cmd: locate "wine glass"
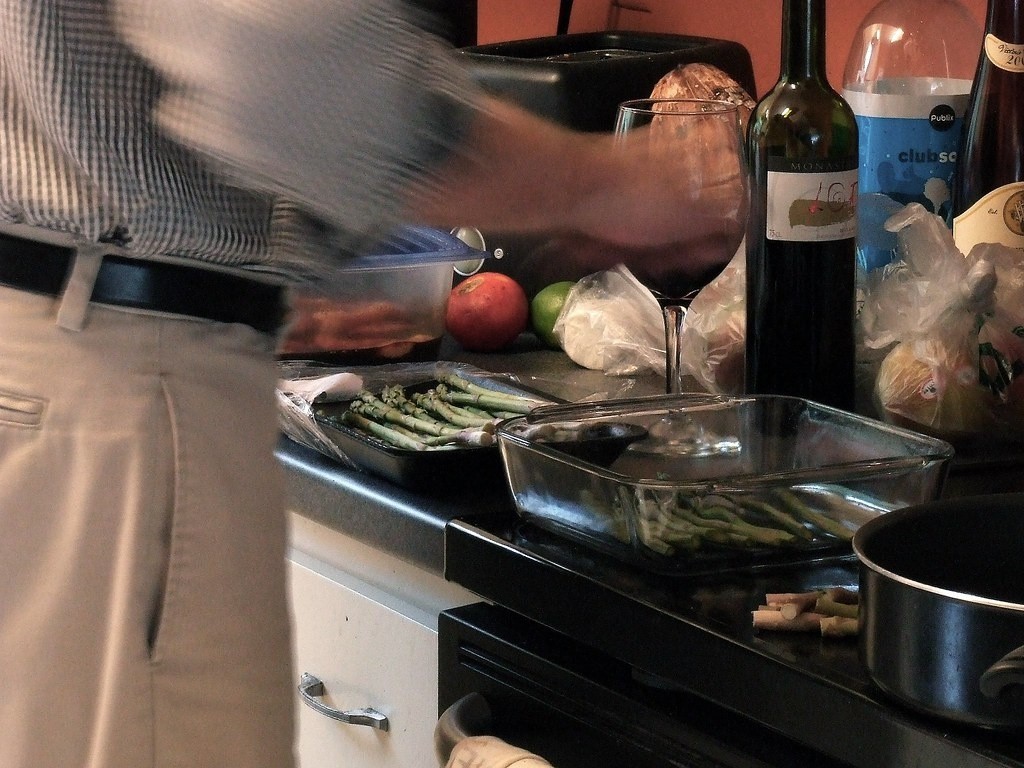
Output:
[608,98,749,460]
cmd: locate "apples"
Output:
[441,272,528,351]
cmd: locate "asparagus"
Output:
[340,373,610,453]
[752,587,861,637]
[575,485,855,555]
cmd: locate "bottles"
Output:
[837,0,994,368]
[745,0,860,440]
[951,0,1024,236]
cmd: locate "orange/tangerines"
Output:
[530,281,580,348]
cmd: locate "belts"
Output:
[0,231,287,334]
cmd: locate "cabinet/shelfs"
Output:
[285,549,437,766]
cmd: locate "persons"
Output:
[0,0,748,768]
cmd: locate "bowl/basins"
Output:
[851,492,1024,734]
